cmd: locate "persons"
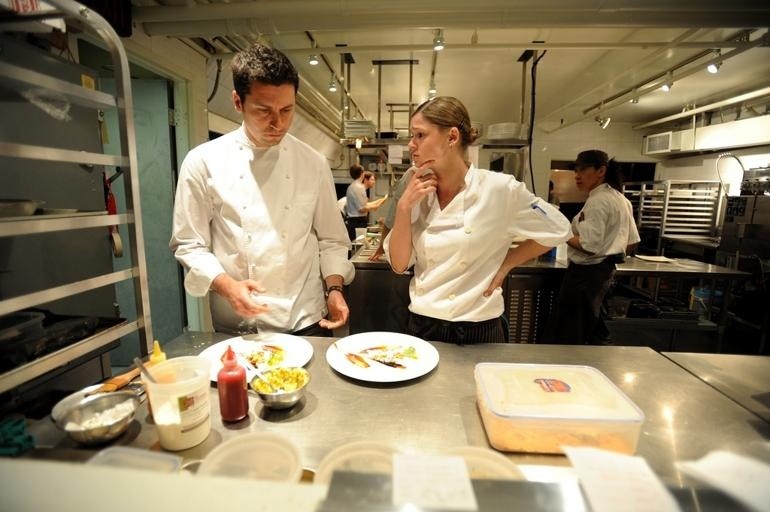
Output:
[166,43,356,341]
[336,163,390,241]
[549,150,641,345]
[382,95,575,345]
[548,179,561,211]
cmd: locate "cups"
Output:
[315,441,412,484]
[196,431,304,484]
[443,445,527,480]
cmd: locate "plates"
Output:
[42,208,79,215]
[51,384,148,422]
[635,254,675,262]
[196,333,314,384]
[325,331,441,382]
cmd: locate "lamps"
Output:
[309,41,338,94]
[592,115,612,131]
[434,27,447,51]
[662,50,728,91]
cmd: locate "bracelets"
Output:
[325,286,343,297]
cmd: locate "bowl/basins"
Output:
[249,366,311,410]
[1,198,46,217]
[57,391,141,446]
[364,226,384,241]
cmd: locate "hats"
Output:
[563,150,608,170]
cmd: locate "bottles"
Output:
[216,344,248,422]
[145,340,168,419]
